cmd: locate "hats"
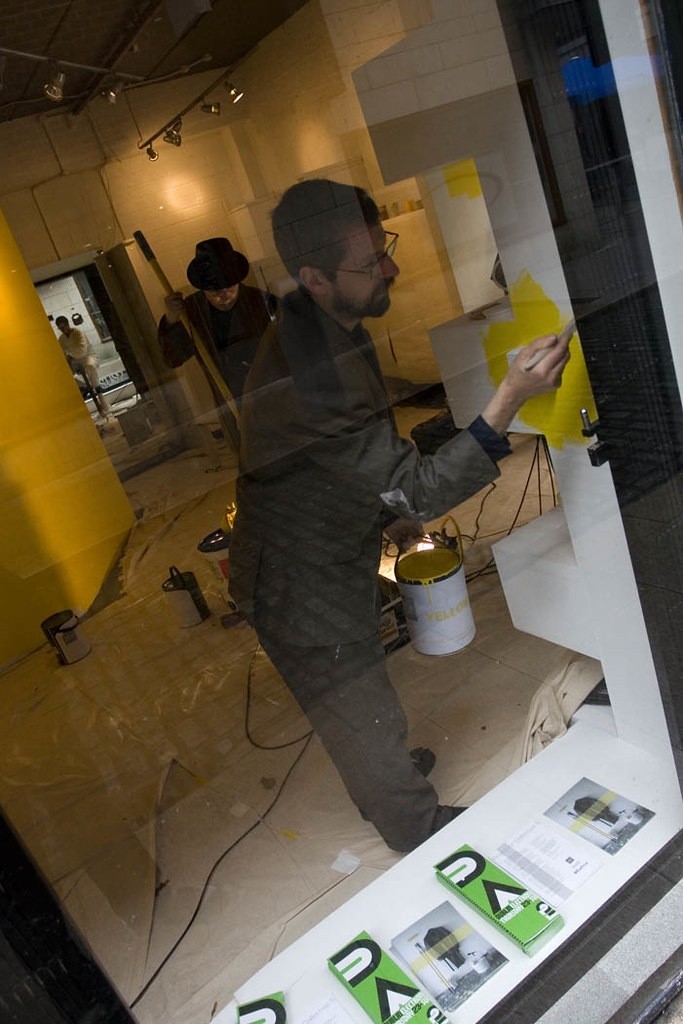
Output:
[186,237,250,292]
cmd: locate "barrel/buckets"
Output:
[197,528,238,614]
[162,565,210,628]
[40,609,94,665]
[395,534,475,657]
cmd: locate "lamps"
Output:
[41,59,66,103]
[102,77,123,104]
[122,45,259,161]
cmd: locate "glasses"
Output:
[321,230,399,280]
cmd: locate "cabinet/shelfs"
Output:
[354,0,683,795]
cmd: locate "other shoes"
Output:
[358,746,436,821]
[98,394,110,412]
[75,379,86,388]
[449,806,469,821]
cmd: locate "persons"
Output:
[54,316,104,395]
[157,238,281,451]
[227,177,573,852]
